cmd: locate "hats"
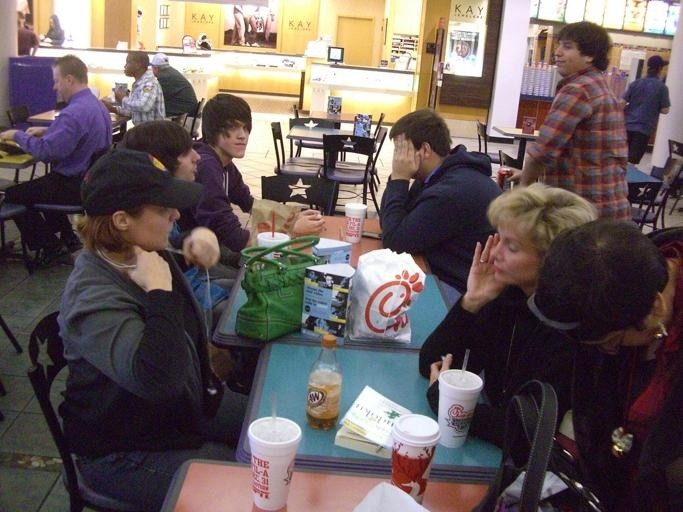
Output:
[146,53,169,66]
[80,148,206,216]
[648,55,669,66]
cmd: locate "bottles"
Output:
[306,334,344,430]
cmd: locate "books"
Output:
[334,383,421,464]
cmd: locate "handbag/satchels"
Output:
[236,236,324,343]
[470,379,604,511]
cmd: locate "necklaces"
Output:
[94,245,215,378]
[610,344,657,463]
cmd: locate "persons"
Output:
[38,14,65,46]
[230,4,248,47]
[376,107,505,293]
[137,10,143,33]
[148,53,201,136]
[17,10,39,57]
[99,50,167,126]
[415,180,600,452]
[523,215,683,511]
[245,12,276,48]
[121,118,259,395]
[166,93,326,387]
[506,20,632,223]
[57,145,236,512]
[620,55,671,167]
[0,52,115,272]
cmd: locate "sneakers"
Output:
[59,233,83,252]
[26,241,70,270]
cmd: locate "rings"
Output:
[480,260,487,263]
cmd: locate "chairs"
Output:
[271,104,388,218]
[627,179,664,230]
[635,165,683,228]
[666,139,683,167]
[26,312,149,511]
[171,97,206,140]
[5,105,33,129]
[262,175,339,215]
[32,143,118,262]
[499,150,522,172]
[0,197,30,352]
[475,119,499,164]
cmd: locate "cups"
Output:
[438,368,485,449]
[246,416,302,511]
[344,202,367,244]
[391,414,442,505]
[257,231,291,262]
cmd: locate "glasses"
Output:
[595,333,626,355]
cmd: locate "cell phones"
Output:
[362,230,383,240]
